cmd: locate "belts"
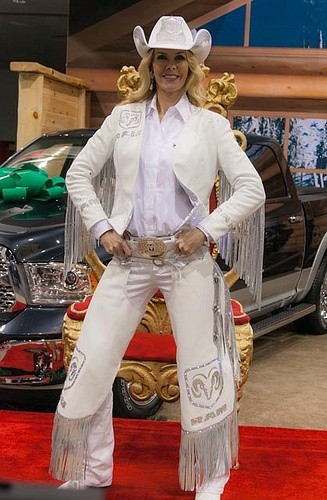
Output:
[125,239,177,258]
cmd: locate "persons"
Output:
[52,13,267,500]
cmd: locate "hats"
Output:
[133,16,212,60]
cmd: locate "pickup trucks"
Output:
[1,127,327,419]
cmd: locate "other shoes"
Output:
[195,492,220,500]
[57,478,91,492]
